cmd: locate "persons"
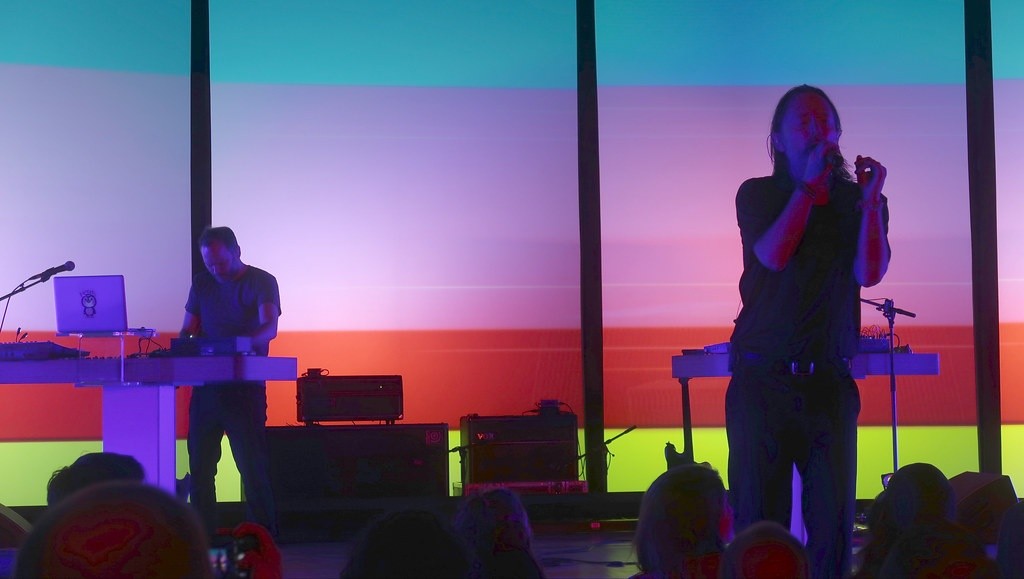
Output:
[629,464,811,579]
[854,463,1024,579]
[727,86,891,579]
[343,489,546,579]
[16,452,282,579]
[184,227,280,536]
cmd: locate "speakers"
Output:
[947,472,1020,544]
[266,422,449,546]
[460,415,579,488]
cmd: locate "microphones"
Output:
[826,151,844,167]
[30,261,75,280]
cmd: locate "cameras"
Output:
[208,534,258,579]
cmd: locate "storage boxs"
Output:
[238,423,448,543]
[459,413,579,482]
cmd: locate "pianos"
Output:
[0,337,297,359]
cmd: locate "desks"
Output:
[0,357,298,496]
[672,352,940,464]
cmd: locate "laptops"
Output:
[53,275,157,336]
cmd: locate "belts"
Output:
[729,356,852,378]
